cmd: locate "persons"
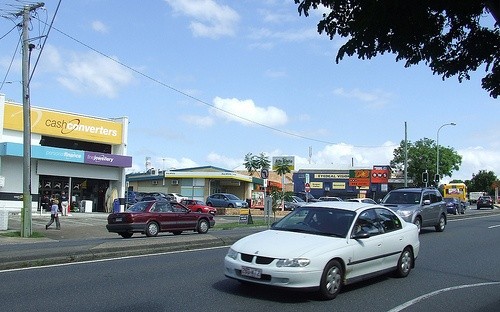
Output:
[45,200,61,230]
[60,191,69,216]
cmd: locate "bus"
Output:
[443,183,467,208]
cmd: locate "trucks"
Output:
[469,192,487,202]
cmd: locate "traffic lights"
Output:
[422,172,428,183]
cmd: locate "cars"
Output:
[128,190,188,207]
[205,193,249,209]
[347,197,378,205]
[223,200,421,300]
[285,195,305,211]
[106,202,215,240]
[443,197,467,215]
[316,196,343,202]
[176,199,216,216]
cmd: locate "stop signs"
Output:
[304,183,311,192]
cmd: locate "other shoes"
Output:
[46,225,48,230]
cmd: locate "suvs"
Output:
[379,186,447,235]
[476,196,494,210]
[284,191,314,202]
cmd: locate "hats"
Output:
[53,200,59,204]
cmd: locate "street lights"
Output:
[436,122,457,189]
[21,42,35,239]
